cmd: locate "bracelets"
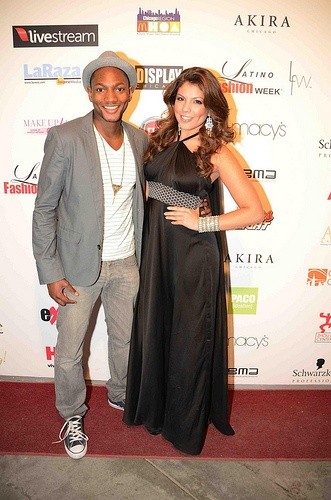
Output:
[198,216,220,233]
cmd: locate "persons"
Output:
[121,67,264,456]
[33,51,148,460]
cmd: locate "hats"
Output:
[82,51,137,94]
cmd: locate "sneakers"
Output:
[108,398,125,411]
[52,415,89,459]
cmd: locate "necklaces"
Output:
[98,132,125,205]
